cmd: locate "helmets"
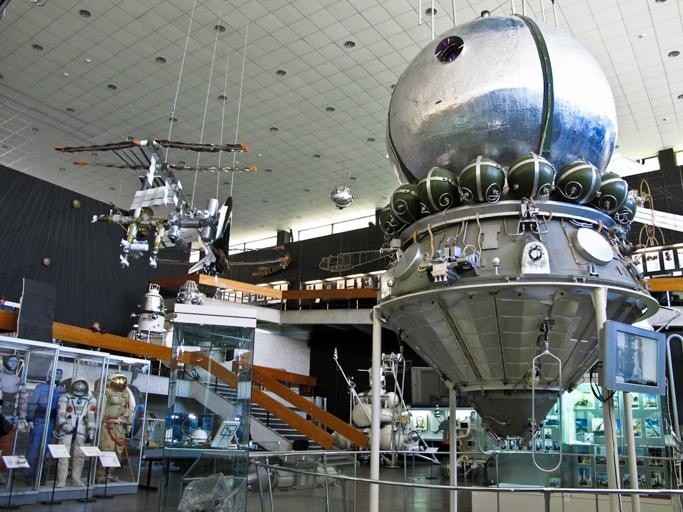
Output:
[107,373,128,392]
[49,367,89,397]
[3,355,18,370]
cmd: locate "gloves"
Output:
[59,422,73,434]
[88,428,95,440]
[18,419,33,432]
[125,425,132,437]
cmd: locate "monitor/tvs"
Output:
[604,320,666,394]
[410,366,473,407]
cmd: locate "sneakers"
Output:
[54,480,85,487]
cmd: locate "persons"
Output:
[461,417,468,423]
[0,355,134,488]
[470,412,475,420]
[416,416,424,428]
[76,322,109,350]
[0,297,6,309]
